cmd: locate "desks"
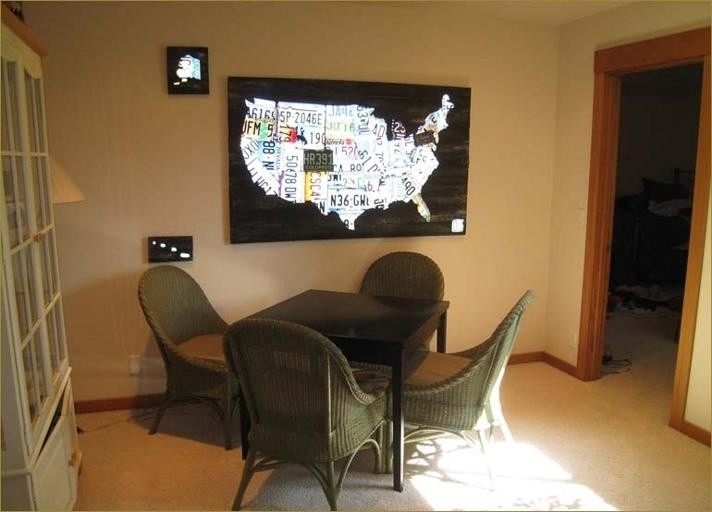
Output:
[230,289,450,492]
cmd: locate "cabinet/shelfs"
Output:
[0,3,83,511]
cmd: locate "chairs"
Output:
[345,252,443,371]
[138,266,240,449]
[380,288,532,474]
[222,318,387,511]
